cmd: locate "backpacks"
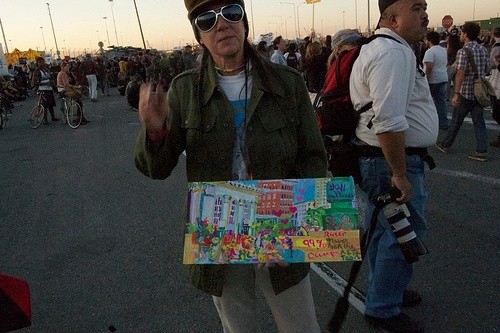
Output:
[313,35,402,135]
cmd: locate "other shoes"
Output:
[43,122,51,126]
[435,140,449,154]
[364,308,421,333]
[402,290,422,305]
[51,117,61,122]
[466,152,488,162]
[75,117,90,125]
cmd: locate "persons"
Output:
[348,0,440,333]
[132,0,328,333]
[0,21,500,162]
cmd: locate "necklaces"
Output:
[212,65,245,72]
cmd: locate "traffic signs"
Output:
[442,15,453,29]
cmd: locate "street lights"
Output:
[40,26,47,52]
[96,30,101,41]
[103,16,110,46]
[9,39,14,52]
[47,2,60,57]
[108,0,119,47]
[264,0,371,40]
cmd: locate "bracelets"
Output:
[147,122,165,137]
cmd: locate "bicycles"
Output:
[61,97,83,130]
[28,90,47,130]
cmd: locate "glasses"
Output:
[195,4,245,33]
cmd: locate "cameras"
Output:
[373,186,429,265]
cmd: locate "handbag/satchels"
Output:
[473,77,491,108]
